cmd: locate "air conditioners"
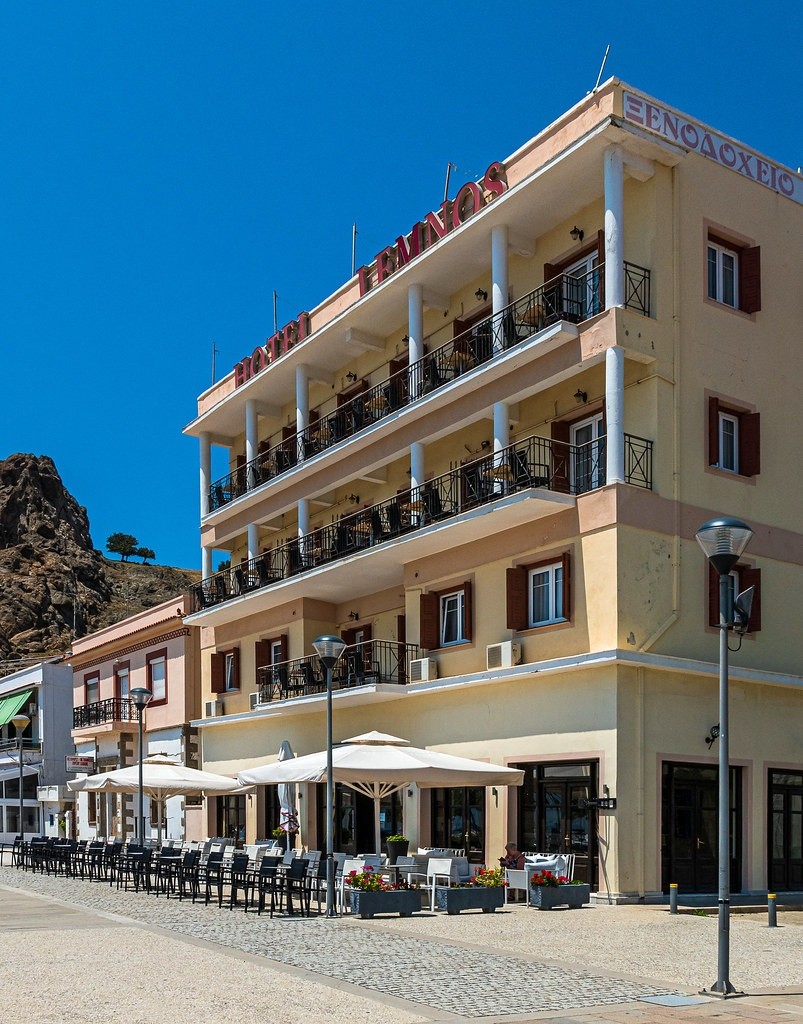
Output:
[205,640,522,738]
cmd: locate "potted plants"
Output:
[273,827,296,854]
[386,834,409,865]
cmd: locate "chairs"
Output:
[0,836,559,919]
[214,285,575,701]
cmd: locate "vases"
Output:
[350,883,590,914]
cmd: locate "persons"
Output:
[499,842,525,901]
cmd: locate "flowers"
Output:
[346,866,582,893]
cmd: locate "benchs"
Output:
[423,847,575,882]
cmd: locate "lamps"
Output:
[348,493,359,504]
[705,723,720,751]
[346,371,357,382]
[114,658,121,666]
[603,785,609,793]
[402,335,409,346]
[574,389,587,403]
[348,611,359,621]
[475,288,487,301]
[570,226,584,242]
[406,467,411,478]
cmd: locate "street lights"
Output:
[130,688,154,848]
[11,715,31,840]
[312,635,347,921]
[697,518,756,1002]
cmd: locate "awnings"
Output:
[0,690,33,726]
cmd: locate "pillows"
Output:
[524,861,557,871]
[418,847,432,855]
[538,854,556,861]
[525,853,539,863]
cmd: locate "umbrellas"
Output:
[66,754,258,841]
[277,740,300,851]
[238,730,526,856]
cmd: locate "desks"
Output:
[375,865,421,885]
